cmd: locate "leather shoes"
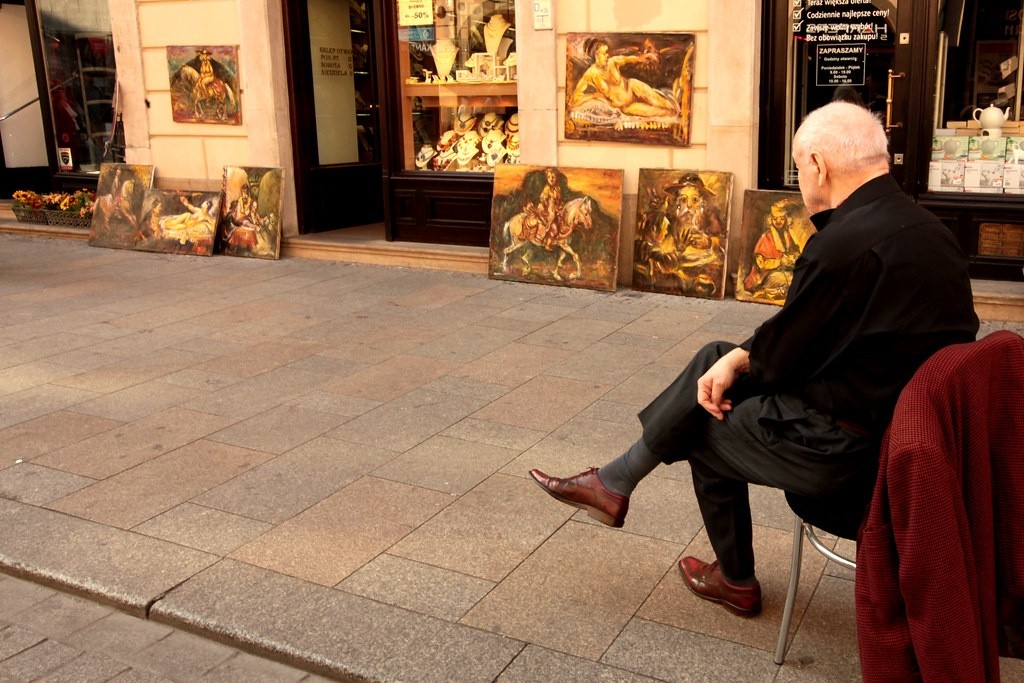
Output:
[528,467,630,528]
[679,556,762,618]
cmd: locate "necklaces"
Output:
[454,112,477,132]
[485,19,508,41]
[505,117,519,133]
[416,131,479,171]
[484,132,521,166]
[479,114,503,132]
[464,52,517,67]
[431,42,457,69]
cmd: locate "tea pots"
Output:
[973,104,1010,129]
[942,138,961,154]
[978,138,999,154]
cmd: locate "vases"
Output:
[11,207,48,225]
[44,209,92,228]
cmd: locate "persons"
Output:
[522,99,980,620]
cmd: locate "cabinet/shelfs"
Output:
[76,31,125,169]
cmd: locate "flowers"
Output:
[13,188,97,217]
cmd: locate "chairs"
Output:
[772,329,1024,683]
